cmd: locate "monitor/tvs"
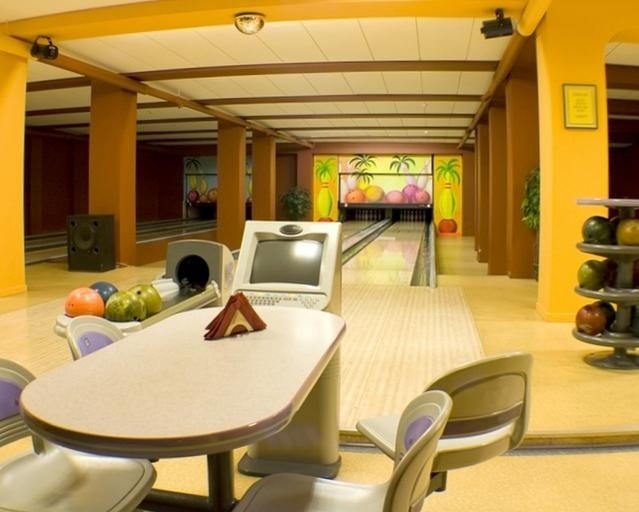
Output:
[249,238,325,286]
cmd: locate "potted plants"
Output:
[521,163,539,281]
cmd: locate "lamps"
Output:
[234,14,264,37]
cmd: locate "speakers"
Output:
[66,213,117,272]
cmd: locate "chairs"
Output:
[355,352,532,496]
[235,387,454,512]
[66,315,123,362]
[0,358,157,511]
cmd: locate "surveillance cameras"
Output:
[30,44,58,60]
[480,16,514,39]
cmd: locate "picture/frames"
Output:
[563,82,598,128]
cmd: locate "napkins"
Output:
[204,291,269,340]
